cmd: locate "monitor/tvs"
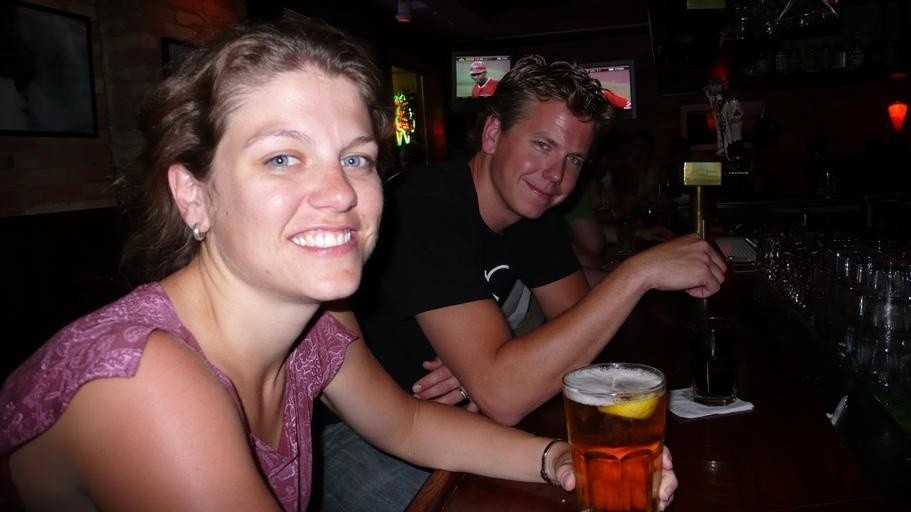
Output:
[571,59,637,121]
[452,52,513,101]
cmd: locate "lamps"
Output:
[395,0,415,23]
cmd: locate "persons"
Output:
[314,57,727,510]
[0,26,679,512]
[469,60,498,96]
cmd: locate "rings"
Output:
[456,387,468,397]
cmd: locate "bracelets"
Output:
[539,437,563,489]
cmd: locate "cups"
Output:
[562,360,666,511]
[835,246,910,371]
[692,316,738,405]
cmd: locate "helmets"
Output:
[468,60,487,75]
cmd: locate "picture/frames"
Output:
[0,0,104,146]
